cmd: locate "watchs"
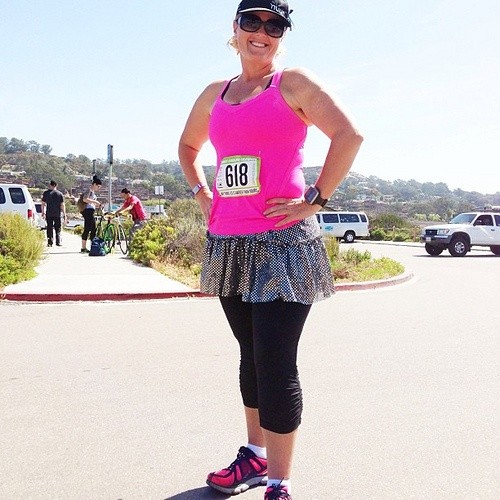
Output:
[304,185,327,209]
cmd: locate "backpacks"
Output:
[88,237,105,256]
[77,189,92,212]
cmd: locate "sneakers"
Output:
[206,446,268,494]
[264,485,293,500]
[81,249,90,253]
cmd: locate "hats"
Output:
[50,180,57,186]
[236,0,293,29]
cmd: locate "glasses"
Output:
[237,13,288,38]
[98,186,100,188]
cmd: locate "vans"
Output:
[33,199,50,230]
[0,184,37,228]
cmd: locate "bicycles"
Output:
[96,210,129,255]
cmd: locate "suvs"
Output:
[313,212,370,243]
[419,210,500,260]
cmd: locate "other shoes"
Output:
[56,243,63,246]
[48,244,52,247]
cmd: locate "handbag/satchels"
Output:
[98,206,105,215]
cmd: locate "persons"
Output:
[115,188,148,238]
[42,180,67,246]
[179,0,364,500]
[77,175,102,253]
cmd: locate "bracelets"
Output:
[192,182,206,195]
